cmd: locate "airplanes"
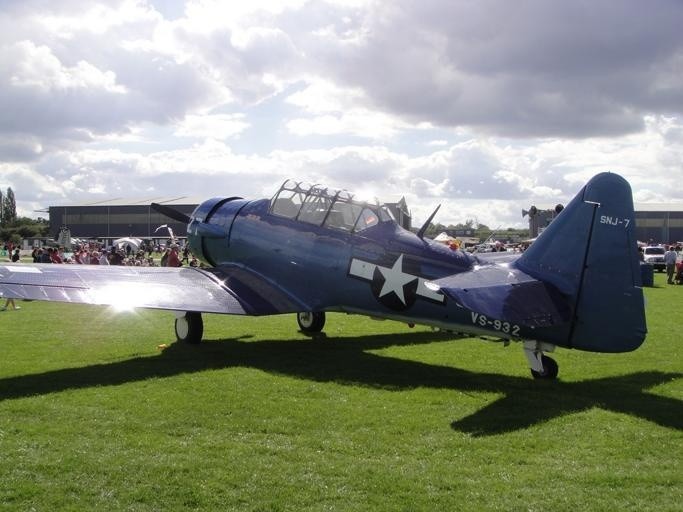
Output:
[0,172,653,378]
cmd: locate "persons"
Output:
[0,298,21,311]
[0,236,200,267]
[663,247,677,284]
[638,246,646,262]
[491,241,507,252]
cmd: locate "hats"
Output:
[171,243,180,249]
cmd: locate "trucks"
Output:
[641,247,667,271]
